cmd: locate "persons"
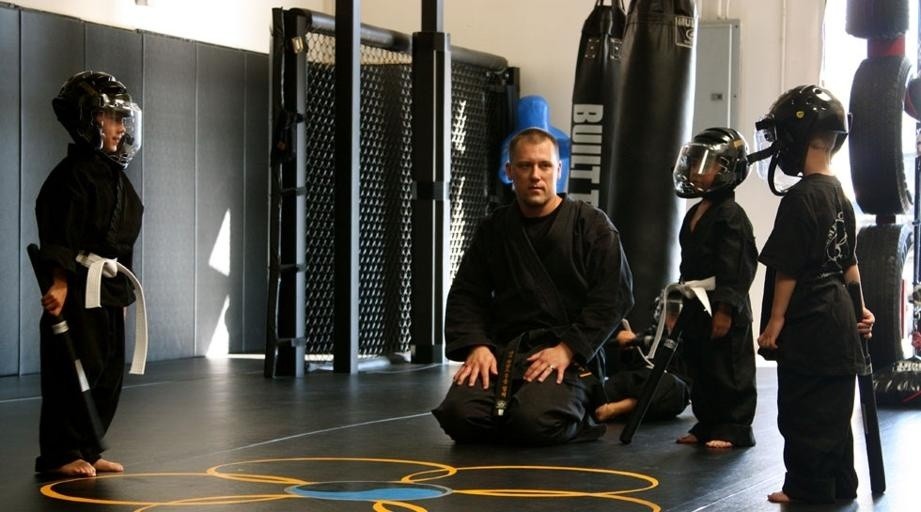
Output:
[754,84,875,504]
[431,127,636,450]
[35,74,148,479]
[594,288,690,423]
[673,127,758,449]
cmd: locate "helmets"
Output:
[50,70,141,168]
[673,127,752,199]
[756,84,847,176]
